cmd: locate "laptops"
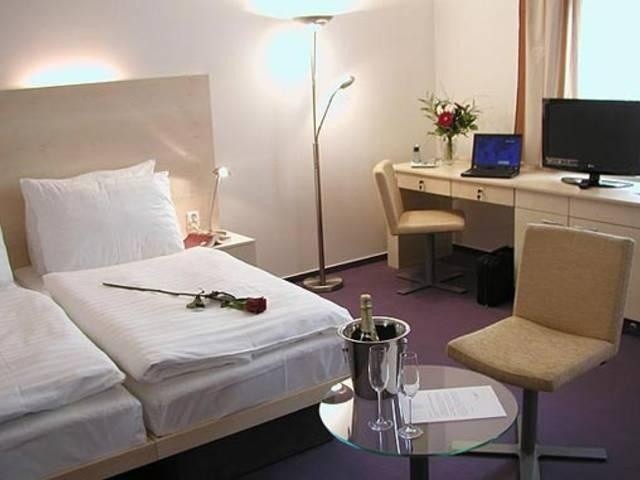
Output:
[461,133,522,178]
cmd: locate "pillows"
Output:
[0,159,183,283]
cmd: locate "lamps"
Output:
[209,166,232,235]
[249,0,355,293]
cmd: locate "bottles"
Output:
[412,144,422,164]
[355,294,379,341]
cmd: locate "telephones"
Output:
[411,145,436,168]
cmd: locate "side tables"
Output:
[319,365,519,480]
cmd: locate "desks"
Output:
[387,159,640,333]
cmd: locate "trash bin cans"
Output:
[477,246,514,308]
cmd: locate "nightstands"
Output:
[183,229,256,266]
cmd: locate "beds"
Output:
[1,274,157,480]
[2,75,353,480]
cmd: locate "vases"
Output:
[440,142,458,164]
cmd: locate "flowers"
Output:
[416,90,482,141]
[102,284,266,314]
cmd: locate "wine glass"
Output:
[368,346,393,432]
[398,352,424,440]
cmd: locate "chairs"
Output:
[373,160,467,295]
[448,223,636,480]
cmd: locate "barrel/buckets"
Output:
[337,318,410,401]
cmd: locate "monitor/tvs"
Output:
[542,98,640,189]
